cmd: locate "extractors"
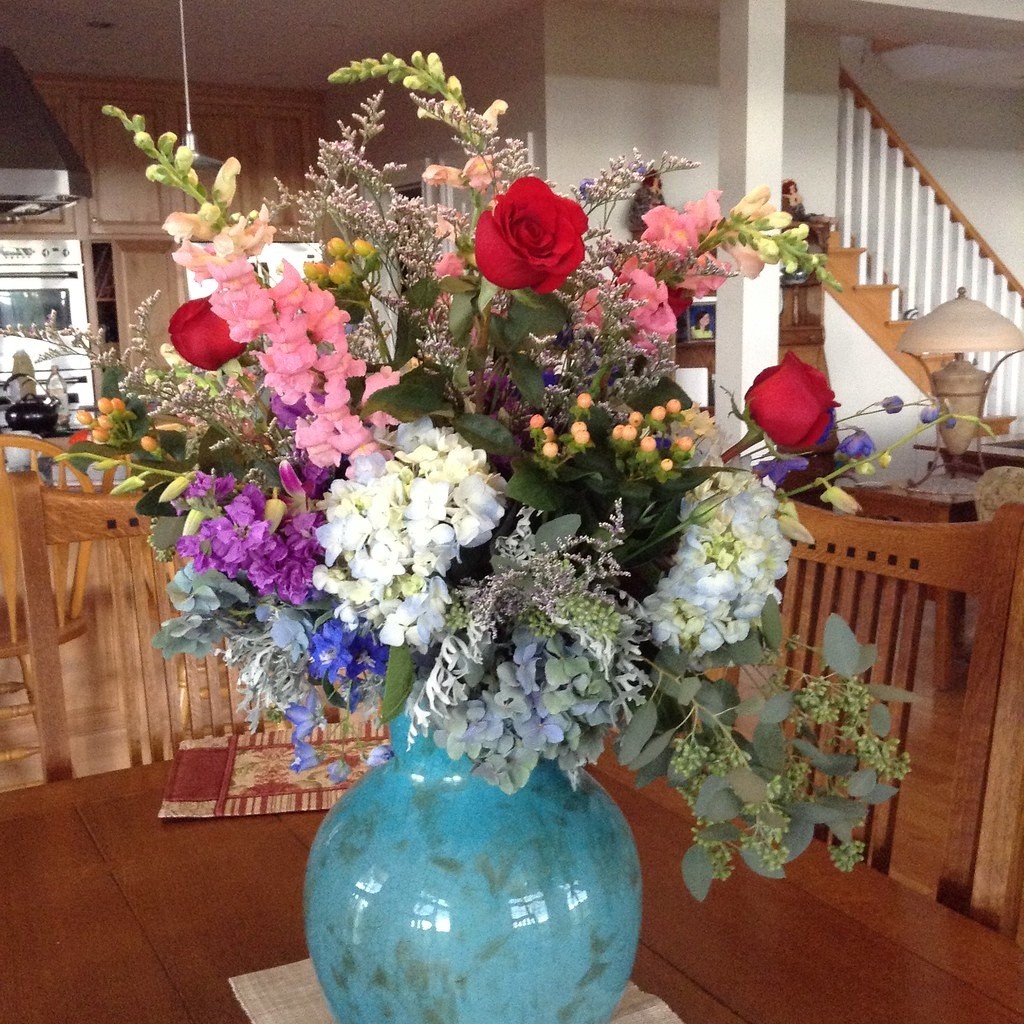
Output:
[1,39,95,223]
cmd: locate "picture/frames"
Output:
[686,298,718,344]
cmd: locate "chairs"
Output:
[767,500,1024,930]
[5,465,264,782]
[0,436,99,778]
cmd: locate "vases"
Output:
[305,704,643,1024]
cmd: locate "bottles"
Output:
[47,364,69,423]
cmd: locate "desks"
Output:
[0,729,1024,1023]
[835,475,984,685]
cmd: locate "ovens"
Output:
[1,235,97,436]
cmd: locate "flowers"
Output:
[0,52,957,790]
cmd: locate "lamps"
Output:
[896,288,1023,489]
[170,0,222,168]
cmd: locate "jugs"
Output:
[4,372,61,432]
